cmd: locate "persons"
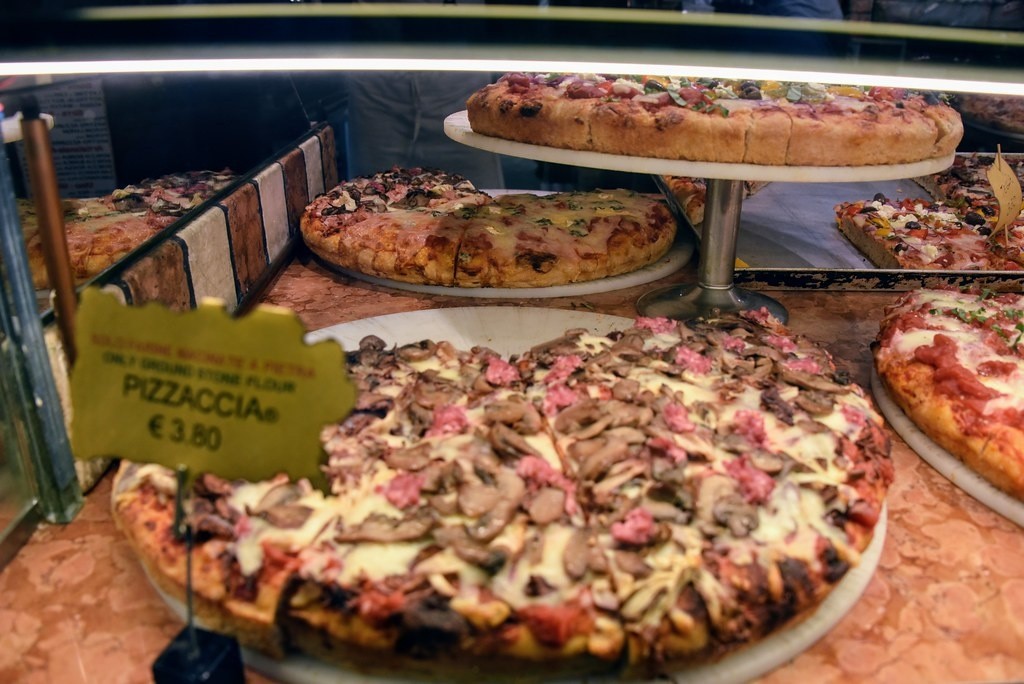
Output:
[325,70,506,189]
[739,0,1024,63]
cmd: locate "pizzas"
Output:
[10,167,245,290]
[833,155,1024,504]
[299,164,774,290]
[115,302,893,680]
[465,70,964,168]
[947,92,1024,134]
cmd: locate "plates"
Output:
[444,108,956,182]
[871,357,1024,530]
[317,189,696,297]
[137,307,888,684]
[652,150,1024,294]
